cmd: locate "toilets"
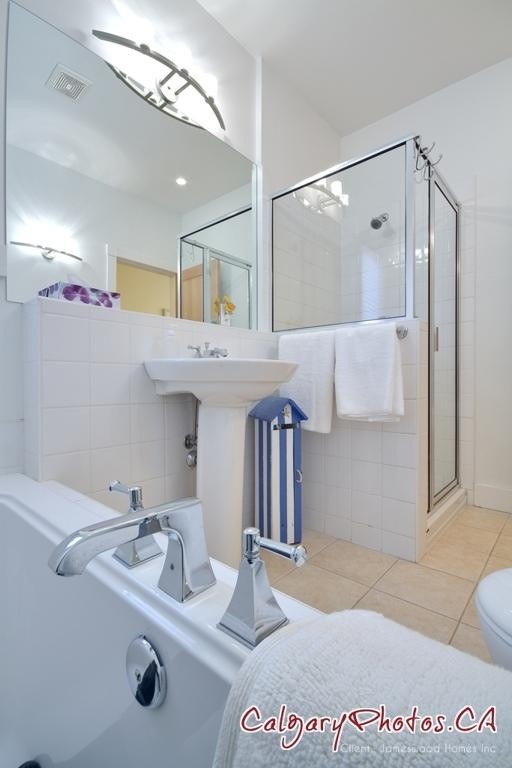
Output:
[471,566,512,673]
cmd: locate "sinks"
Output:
[143,357,299,402]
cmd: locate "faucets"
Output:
[202,341,230,358]
[46,497,218,604]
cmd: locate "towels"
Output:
[334,321,404,424]
[213,608,512,768]
[278,331,335,434]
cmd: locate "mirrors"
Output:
[272,141,407,331]
[6,2,258,333]
[177,203,257,331]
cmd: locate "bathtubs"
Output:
[0,469,326,768]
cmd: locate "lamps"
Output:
[294,178,350,217]
[92,14,227,130]
